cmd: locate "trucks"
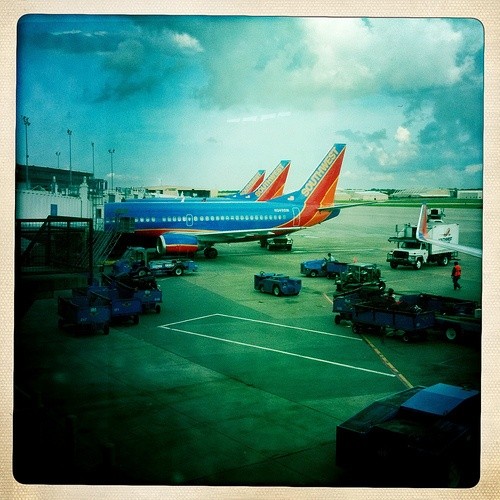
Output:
[386,222,461,271]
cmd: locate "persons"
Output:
[451,262,462,291]
[328,253,335,261]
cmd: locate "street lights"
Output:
[22,115,31,190]
[108,149,115,191]
[91,142,95,173]
[67,128,73,186]
[56,151,62,168]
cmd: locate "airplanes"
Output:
[103,143,348,259]
